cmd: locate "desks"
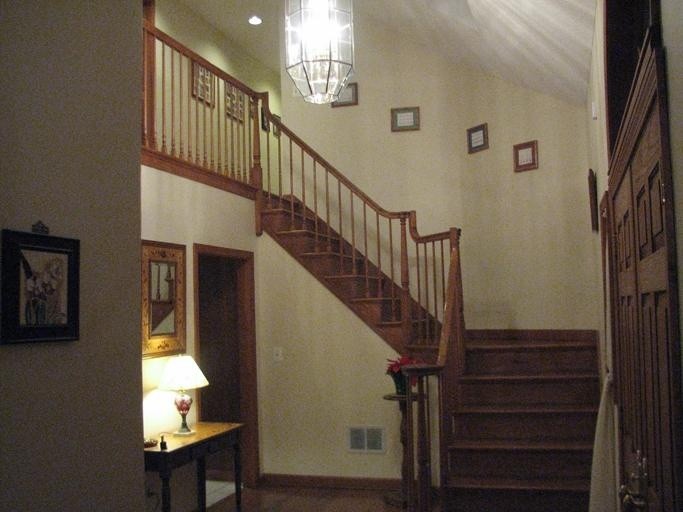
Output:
[383,393,419,505]
[143,421,245,512]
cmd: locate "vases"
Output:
[391,372,412,394]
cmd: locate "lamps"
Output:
[159,354,211,438]
[284,1,355,105]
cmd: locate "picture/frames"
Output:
[190,61,282,137]
[141,238,188,359]
[1,220,82,348]
[512,139,539,173]
[466,121,490,155]
[390,106,420,132]
[330,81,358,108]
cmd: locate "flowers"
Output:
[385,354,424,387]
